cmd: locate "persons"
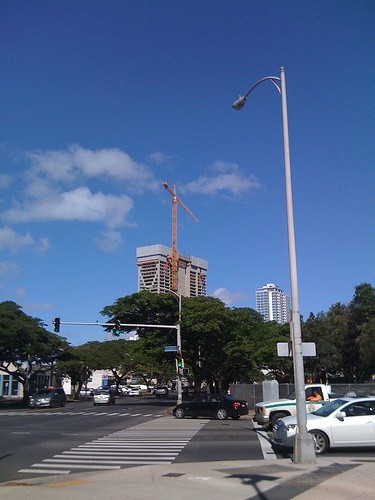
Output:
[307,390,322,402]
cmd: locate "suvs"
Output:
[93,389,113,406]
[154,387,170,398]
[29,388,67,408]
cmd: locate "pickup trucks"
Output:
[251,384,375,455]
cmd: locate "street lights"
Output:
[231,66,320,464]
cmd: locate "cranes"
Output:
[162,181,200,292]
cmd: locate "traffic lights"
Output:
[53,318,60,332]
[115,320,122,336]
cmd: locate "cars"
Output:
[77,385,146,398]
[172,393,249,420]
